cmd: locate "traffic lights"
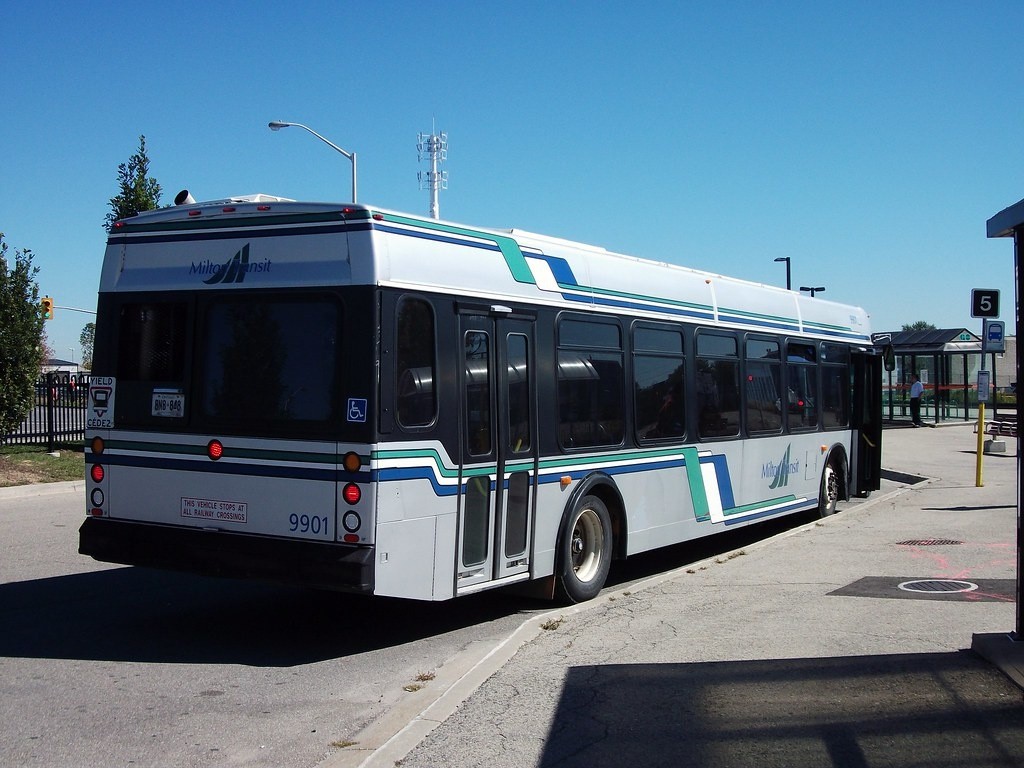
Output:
[40,298,53,320]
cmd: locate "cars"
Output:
[774,385,803,413]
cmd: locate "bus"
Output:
[79,187,894,601]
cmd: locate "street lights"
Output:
[268,120,358,205]
[773,257,791,291]
[800,285,826,298]
[68,347,74,361]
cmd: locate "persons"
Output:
[910,375,924,427]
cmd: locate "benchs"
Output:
[973,414,1018,440]
